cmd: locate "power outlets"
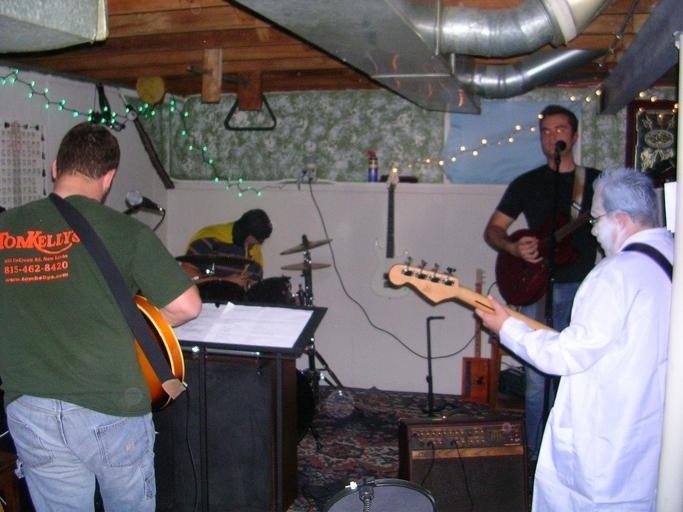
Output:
[299,163,317,184]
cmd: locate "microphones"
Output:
[553,139,567,160]
[126,190,163,213]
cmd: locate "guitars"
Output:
[120,292,193,416]
[493,216,610,310]
[378,255,564,358]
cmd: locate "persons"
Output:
[476,164,676,512]
[483,105,604,496]
[0,122,201,512]
[182,209,273,289]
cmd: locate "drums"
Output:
[196,278,250,308]
[325,477,437,512]
[247,274,297,309]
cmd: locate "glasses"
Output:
[589,209,611,225]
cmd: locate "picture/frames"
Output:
[623,100,678,189]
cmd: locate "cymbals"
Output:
[281,263,332,272]
[173,254,264,270]
[281,239,333,257]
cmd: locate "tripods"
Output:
[273,249,368,449]
